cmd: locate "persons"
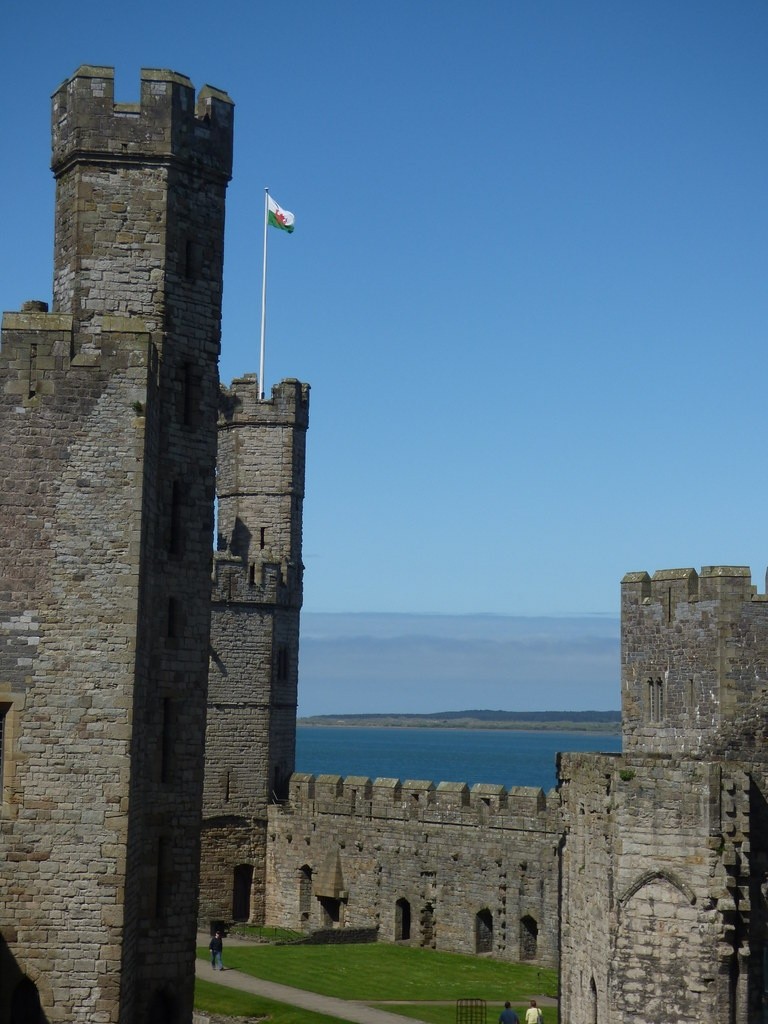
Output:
[209,932,224,971]
[499,1001,519,1024]
[525,1000,544,1024]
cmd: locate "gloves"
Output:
[209,949,212,952]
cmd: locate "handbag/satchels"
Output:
[537,1008,543,1024]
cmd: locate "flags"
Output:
[266,193,295,234]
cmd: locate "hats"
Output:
[216,931,220,934]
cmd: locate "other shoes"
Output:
[212,968,216,970]
[220,967,223,971]
